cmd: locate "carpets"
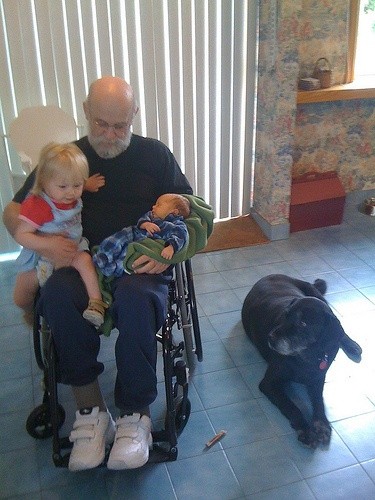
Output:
[198,214,271,253]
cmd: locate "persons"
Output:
[14,144,103,327]
[3,75,193,471]
[93,194,190,275]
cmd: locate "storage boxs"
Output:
[291,175,347,233]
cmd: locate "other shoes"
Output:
[83,298,109,326]
[23,301,35,325]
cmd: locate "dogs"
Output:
[242,273,363,449]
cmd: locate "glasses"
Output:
[89,110,132,129]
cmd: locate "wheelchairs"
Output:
[25,259,205,470]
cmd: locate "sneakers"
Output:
[107,413,153,470]
[69,406,117,472]
